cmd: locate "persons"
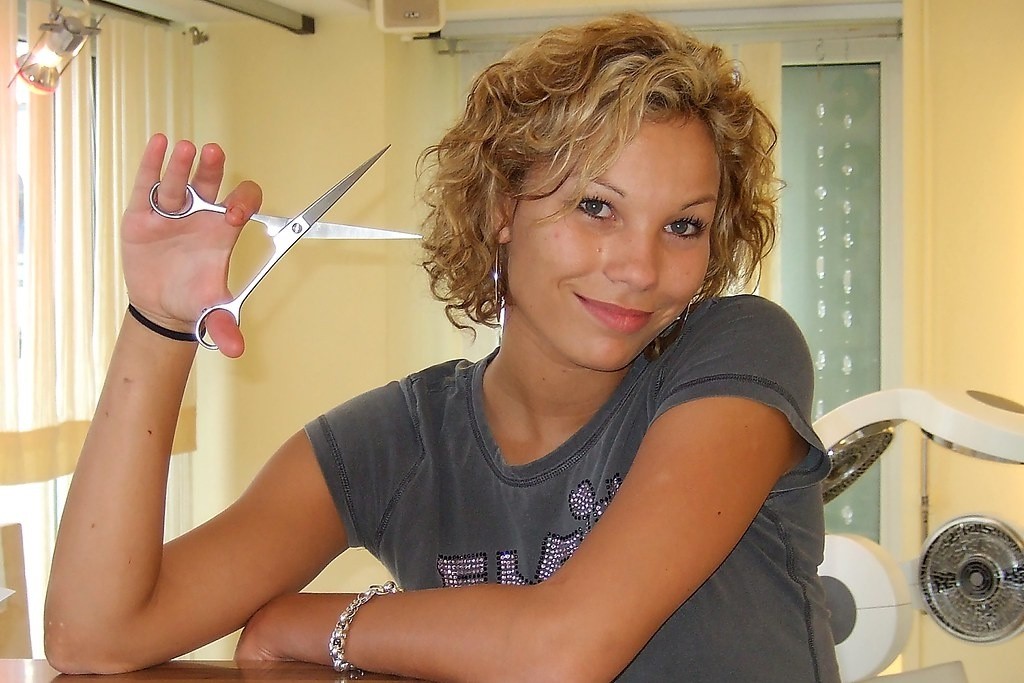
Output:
[44,14,840,683]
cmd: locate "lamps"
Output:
[7,0,106,96]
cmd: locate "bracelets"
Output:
[128,305,205,341]
[328,580,404,673]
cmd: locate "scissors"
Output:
[149,143,426,352]
